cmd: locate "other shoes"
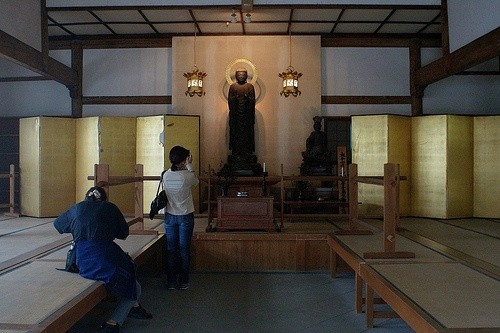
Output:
[127,304,152,320]
[167,281,177,289]
[178,281,192,290]
[96,322,120,333]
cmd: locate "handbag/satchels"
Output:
[155,170,167,211]
[66,249,73,272]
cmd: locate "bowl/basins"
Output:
[238,191,247,195]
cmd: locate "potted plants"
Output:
[291,179,311,203]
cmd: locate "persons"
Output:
[301,117,331,166]
[228,68,256,155]
[53,186,153,332]
[162,146,199,290]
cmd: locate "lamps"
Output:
[182,23,208,99]
[244,7,253,23]
[278,22,304,98]
[230,9,238,24]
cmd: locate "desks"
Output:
[287,201,363,221]
[215,195,277,232]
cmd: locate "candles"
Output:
[341,166,344,176]
[263,161,266,172]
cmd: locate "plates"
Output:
[237,194,248,196]
